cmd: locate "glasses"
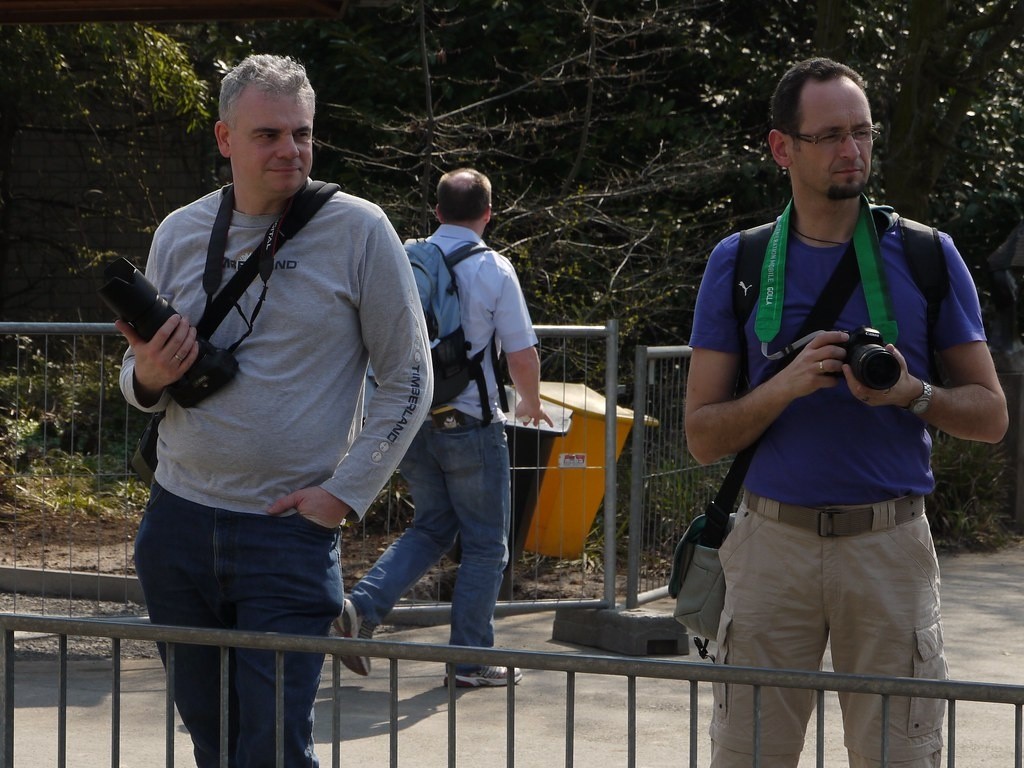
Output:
[790,128,882,144]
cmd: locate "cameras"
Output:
[822,327,901,390]
[95,257,239,408]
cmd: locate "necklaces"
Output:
[789,223,847,245]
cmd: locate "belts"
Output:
[741,492,924,537]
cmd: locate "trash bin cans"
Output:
[443,384,575,581]
[522,379,657,550]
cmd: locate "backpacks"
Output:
[367,238,510,428]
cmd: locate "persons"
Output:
[333,168,554,688]
[685,58,1009,768]
[119,53,434,768]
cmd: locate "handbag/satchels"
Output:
[668,507,736,641]
[131,410,166,482]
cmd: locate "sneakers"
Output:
[444,658,522,688]
[334,596,372,676]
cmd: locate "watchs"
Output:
[898,379,932,414]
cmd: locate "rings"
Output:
[820,361,826,373]
[862,395,870,401]
[175,354,183,362]
